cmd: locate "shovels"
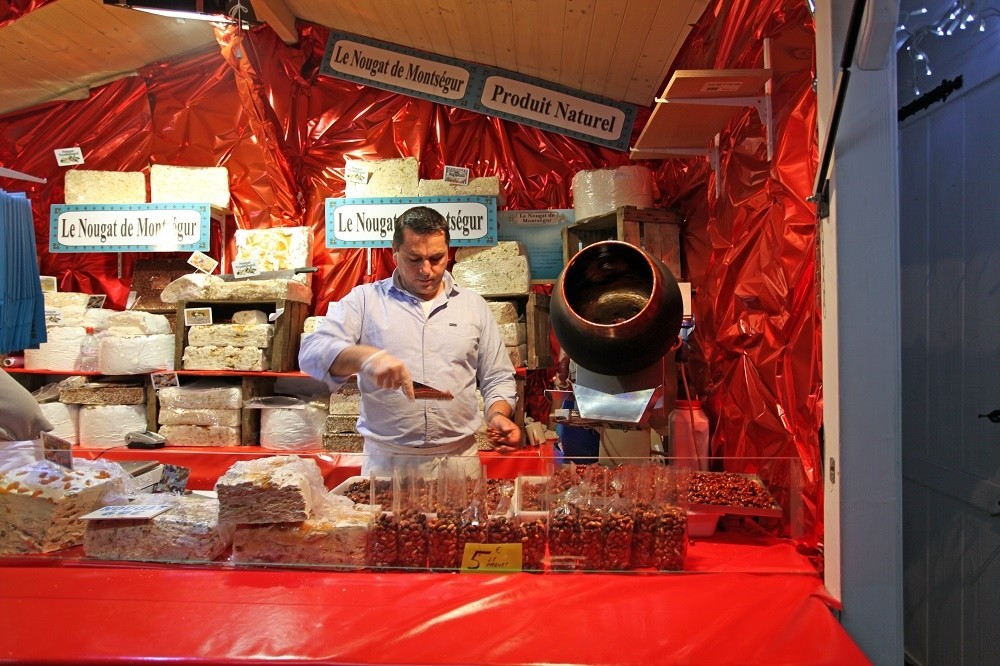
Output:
[402,377,455,400]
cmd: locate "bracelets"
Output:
[488,412,503,423]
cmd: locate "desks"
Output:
[0,531,871,666]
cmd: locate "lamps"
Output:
[104,1,264,27]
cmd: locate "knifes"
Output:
[213,266,320,283]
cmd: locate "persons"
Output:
[297,207,523,481]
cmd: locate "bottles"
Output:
[81,326,100,373]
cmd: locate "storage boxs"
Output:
[595,427,651,467]
